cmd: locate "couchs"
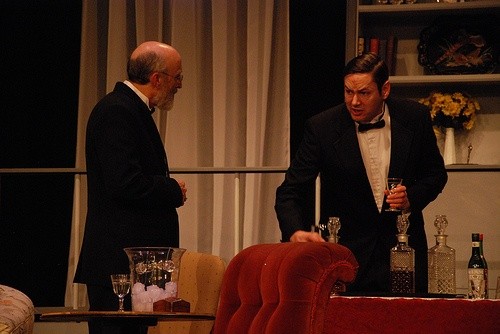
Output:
[145,238,358,334]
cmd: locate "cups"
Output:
[124,246,189,312]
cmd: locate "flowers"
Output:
[418,91,482,135]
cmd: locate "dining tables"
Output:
[38,309,216,334]
[323,291,500,334]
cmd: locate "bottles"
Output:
[467,233,489,298]
[327,217,346,294]
[427,213,457,295]
[389,212,417,296]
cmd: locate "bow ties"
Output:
[359,120,385,132]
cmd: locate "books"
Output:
[358,35,398,78]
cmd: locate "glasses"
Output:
[158,71,183,82]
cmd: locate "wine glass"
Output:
[111,274,131,312]
[384,178,402,212]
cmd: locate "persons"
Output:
[275,54,449,298]
[73,41,189,332]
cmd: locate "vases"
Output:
[440,128,458,167]
